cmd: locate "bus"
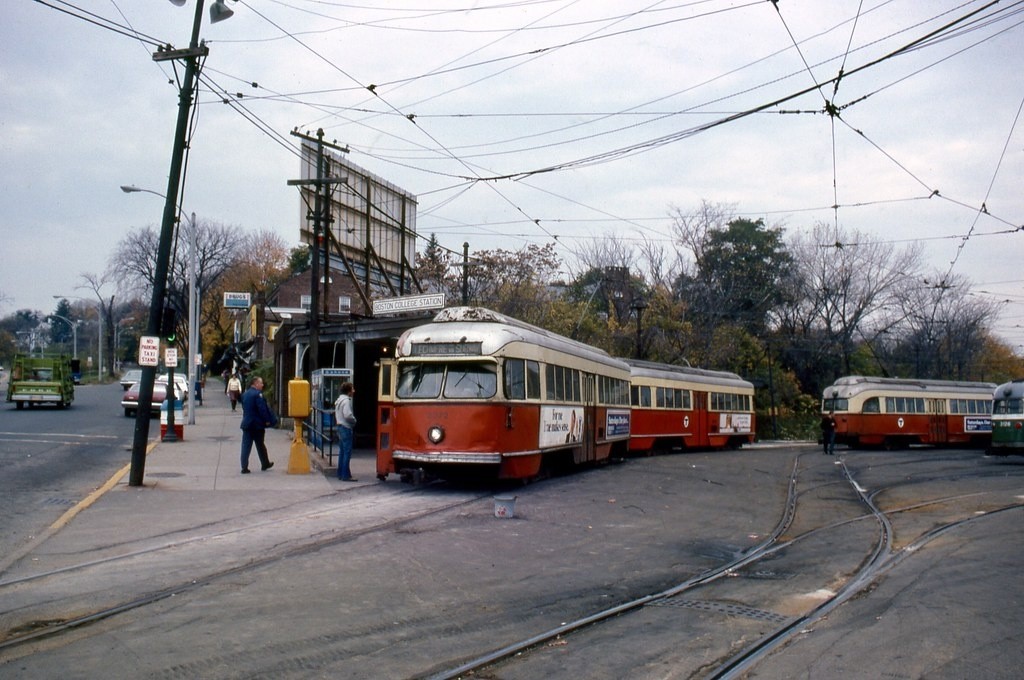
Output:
[989,379,1024,451]
[375,304,756,487]
[820,374,998,447]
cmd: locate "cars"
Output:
[121,383,169,417]
[156,371,188,406]
[120,370,143,390]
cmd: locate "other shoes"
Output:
[340,476,359,482]
[261,462,275,471]
[824,450,834,455]
[240,469,251,474]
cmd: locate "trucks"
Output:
[6,351,75,408]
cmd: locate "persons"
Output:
[334,381,358,482]
[239,375,274,474]
[226,374,242,413]
[820,409,838,455]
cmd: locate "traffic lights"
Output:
[166,309,176,343]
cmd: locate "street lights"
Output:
[33,313,77,360]
[120,183,197,427]
[54,294,102,384]
[15,331,44,360]
